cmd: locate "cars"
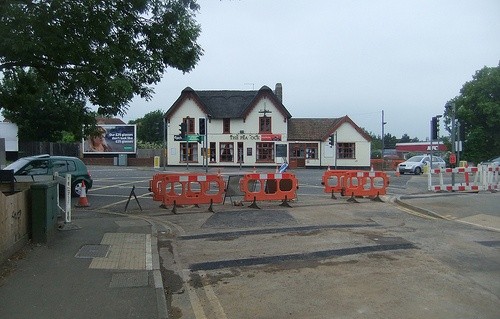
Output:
[398,155,446,176]
[2,154,93,198]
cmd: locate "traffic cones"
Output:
[74,180,92,208]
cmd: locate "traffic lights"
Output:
[432,116,440,140]
[328,133,335,146]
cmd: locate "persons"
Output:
[85,126,113,152]
[448,150,456,169]
